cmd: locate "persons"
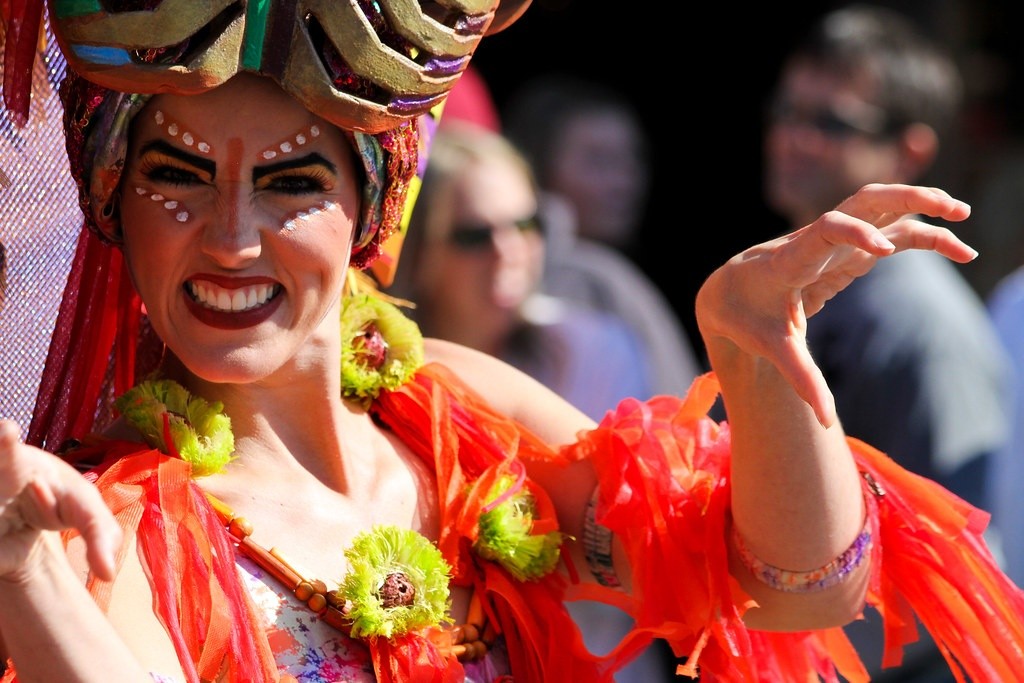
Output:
[388,15,1023,590]
[0,0,1024,683]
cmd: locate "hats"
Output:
[46,0,531,270]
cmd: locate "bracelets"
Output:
[727,455,883,594]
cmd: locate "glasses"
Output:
[771,95,905,141]
[417,210,544,251]
